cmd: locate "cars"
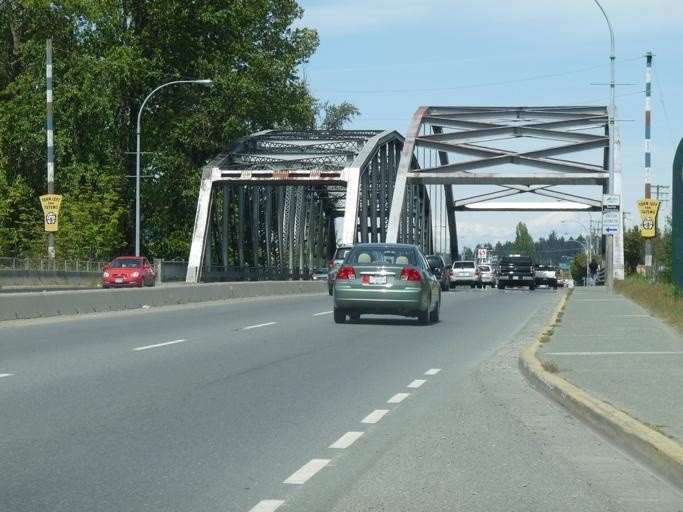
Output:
[328,243,564,325]
[102,256,155,288]
[312,268,328,279]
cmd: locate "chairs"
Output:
[357,252,408,264]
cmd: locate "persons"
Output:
[587,258,600,286]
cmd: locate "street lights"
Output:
[135,80,213,257]
[561,220,591,285]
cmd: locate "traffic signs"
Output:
[602,194,620,235]
